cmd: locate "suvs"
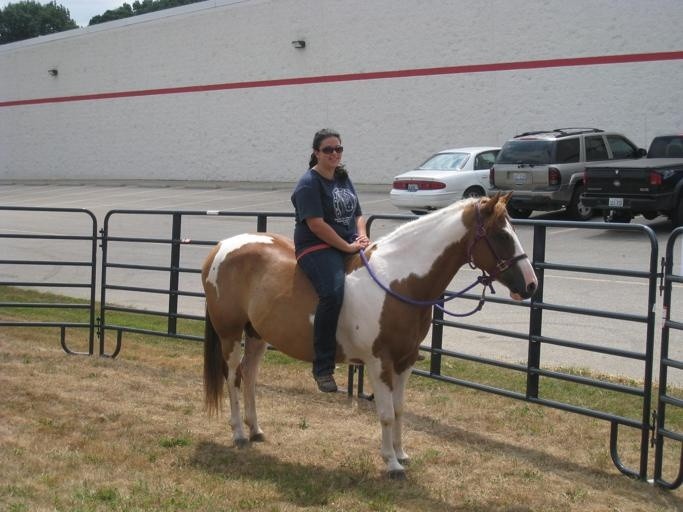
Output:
[482,124,645,221]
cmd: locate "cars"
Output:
[386,145,502,217]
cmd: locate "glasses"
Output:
[318,146,343,154]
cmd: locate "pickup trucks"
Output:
[580,132,682,229]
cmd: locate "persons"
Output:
[290,127,371,393]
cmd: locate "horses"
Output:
[198,191,538,477]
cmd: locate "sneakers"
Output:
[313,375,338,392]
